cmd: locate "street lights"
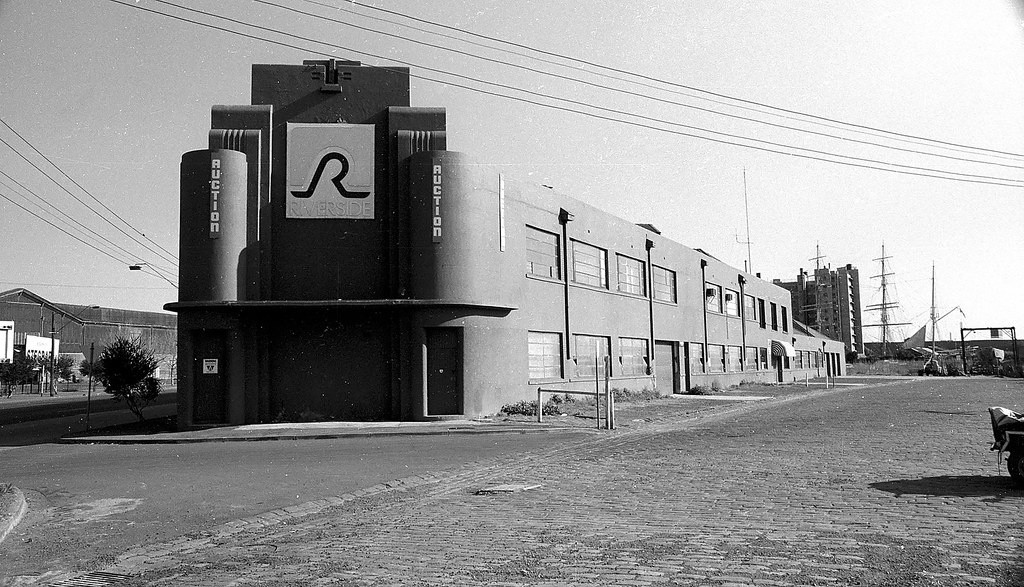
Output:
[50,303,100,397]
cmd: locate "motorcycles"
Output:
[987,404,1024,481]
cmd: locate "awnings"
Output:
[771,339,797,358]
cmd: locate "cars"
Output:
[970,361,994,376]
